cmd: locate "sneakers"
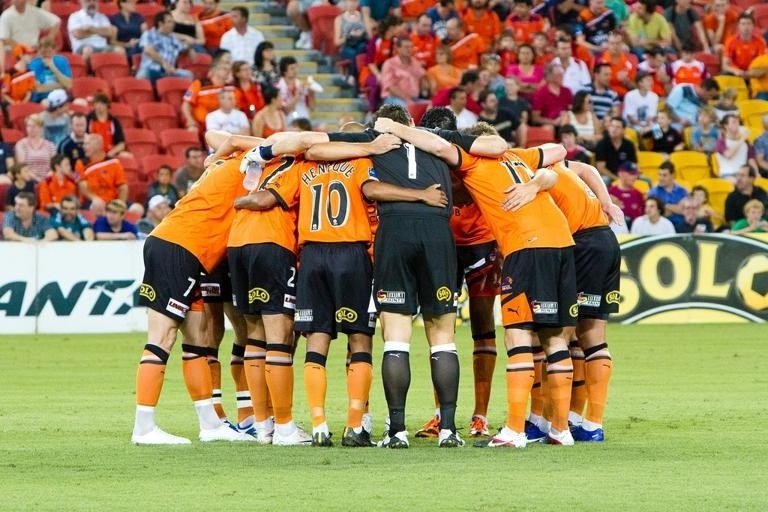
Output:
[438,428,466,448]
[198,414,410,448]
[132,427,191,444]
[498,415,604,446]
[473,425,527,448]
[468,417,490,437]
[415,415,440,438]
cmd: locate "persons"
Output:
[1,1,315,243]
[278,1,768,241]
[130,102,624,449]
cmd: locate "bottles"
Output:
[737,125,751,139]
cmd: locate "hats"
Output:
[46,89,68,112]
[619,161,641,175]
[106,199,127,212]
[149,194,171,209]
